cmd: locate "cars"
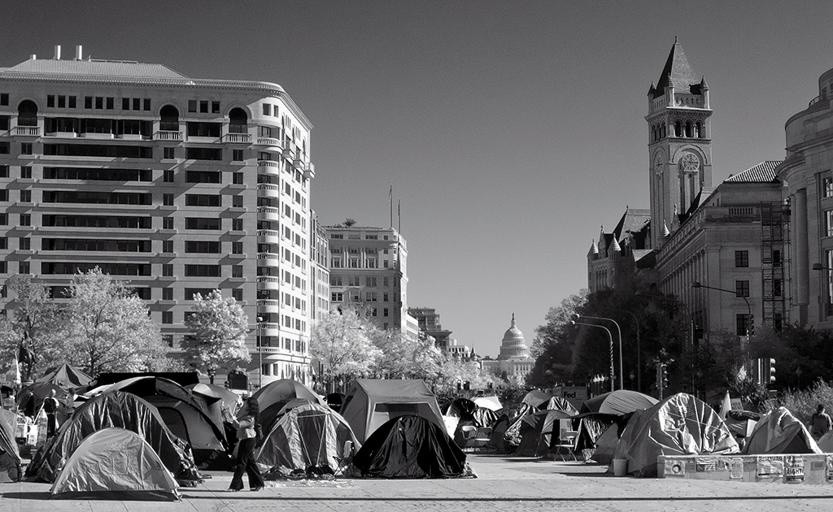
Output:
[484,386,535,401]
[438,397,450,415]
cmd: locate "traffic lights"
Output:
[770,358,775,382]
[663,369,670,389]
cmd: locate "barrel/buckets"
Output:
[612,458,627,477]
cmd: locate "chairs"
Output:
[333,441,355,479]
[553,431,579,462]
[461,425,493,454]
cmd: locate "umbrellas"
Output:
[35,363,94,387]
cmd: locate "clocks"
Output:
[683,153,699,170]
[654,150,665,175]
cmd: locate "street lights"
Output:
[591,373,605,393]
[327,326,365,391]
[690,320,703,395]
[569,320,616,391]
[690,280,752,386]
[573,313,623,390]
[550,356,562,386]
[254,316,263,389]
[812,263,832,272]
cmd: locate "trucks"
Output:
[552,386,587,402]
[477,389,484,397]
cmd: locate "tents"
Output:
[439,389,833,479]
[1,378,479,500]
